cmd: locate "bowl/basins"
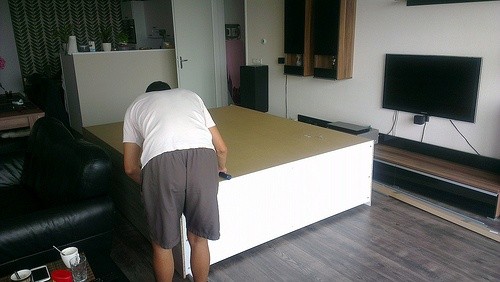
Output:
[11,269,33,282]
[78,45,90,52]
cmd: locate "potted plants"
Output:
[116,32,128,51]
[100,25,112,51]
[55,24,73,51]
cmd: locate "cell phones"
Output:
[30,265,51,282]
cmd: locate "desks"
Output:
[0,93,46,131]
[0,247,129,282]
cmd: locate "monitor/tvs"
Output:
[382,54,483,123]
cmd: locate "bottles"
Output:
[160,35,172,49]
[152,27,159,36]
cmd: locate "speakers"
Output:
[239,64,268,113]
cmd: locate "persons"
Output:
[123,80,227,282]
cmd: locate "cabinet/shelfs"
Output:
[371,143,500,218]
[283,0,357,79]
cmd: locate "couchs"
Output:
[0,117,116,276]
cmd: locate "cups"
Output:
[60,247,80,269]
[102,42,111,51]
[69,255,87,282]
